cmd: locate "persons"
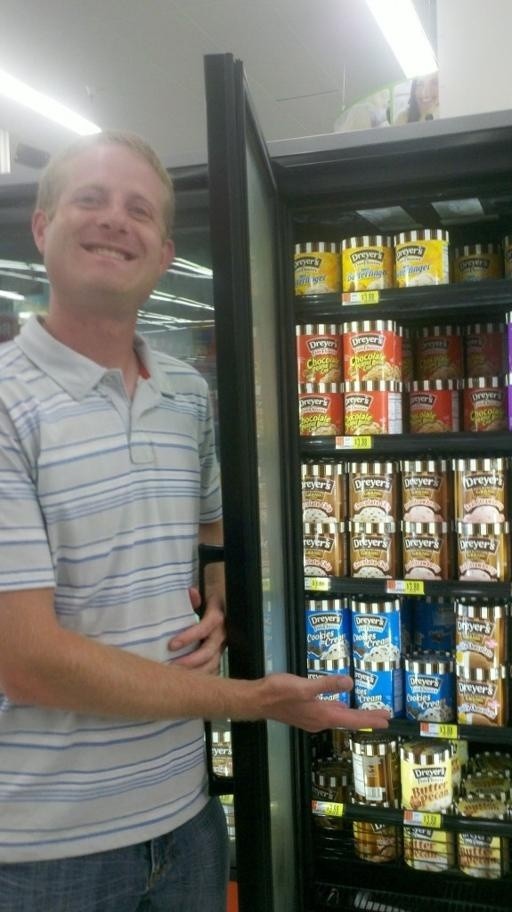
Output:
[2,132,398,912]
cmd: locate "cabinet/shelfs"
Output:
[278,184,512,907]
